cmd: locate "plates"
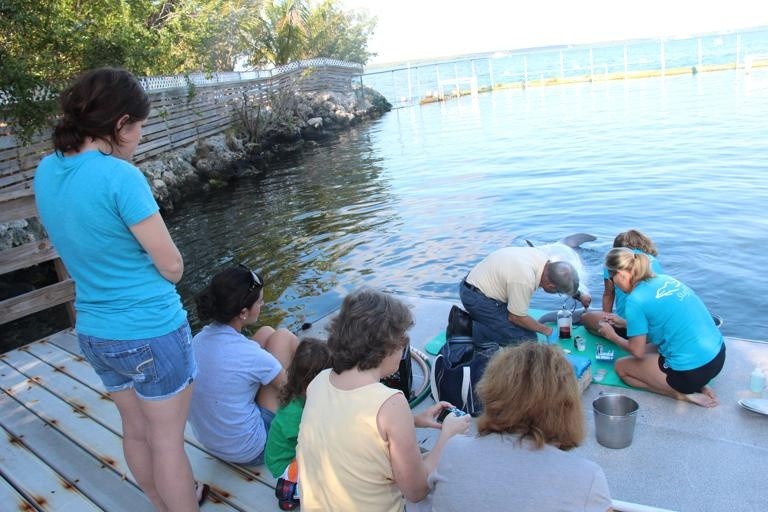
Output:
[737,397,768,415]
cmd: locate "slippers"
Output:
[193,479,210,508]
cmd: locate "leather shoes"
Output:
[446,305,469,340]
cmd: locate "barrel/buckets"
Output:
[592,394,639,449]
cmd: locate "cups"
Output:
[575,336,586,351]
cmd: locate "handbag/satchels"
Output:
[430,336,504,417]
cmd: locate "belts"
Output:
[464,270,488,299]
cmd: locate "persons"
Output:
[425,340,614,511]
[185,262,299,470]
[439,249,591,356]
[32,69,208,512]
[297,288,469,511]
[600,239,725,413]
[582,232,669,333]
[265,339,337,505]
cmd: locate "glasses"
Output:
[238,263,261,306]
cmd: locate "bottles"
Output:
[556,304,572,340]
[748,359,765,392]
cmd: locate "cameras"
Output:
[437,407,466,424]
